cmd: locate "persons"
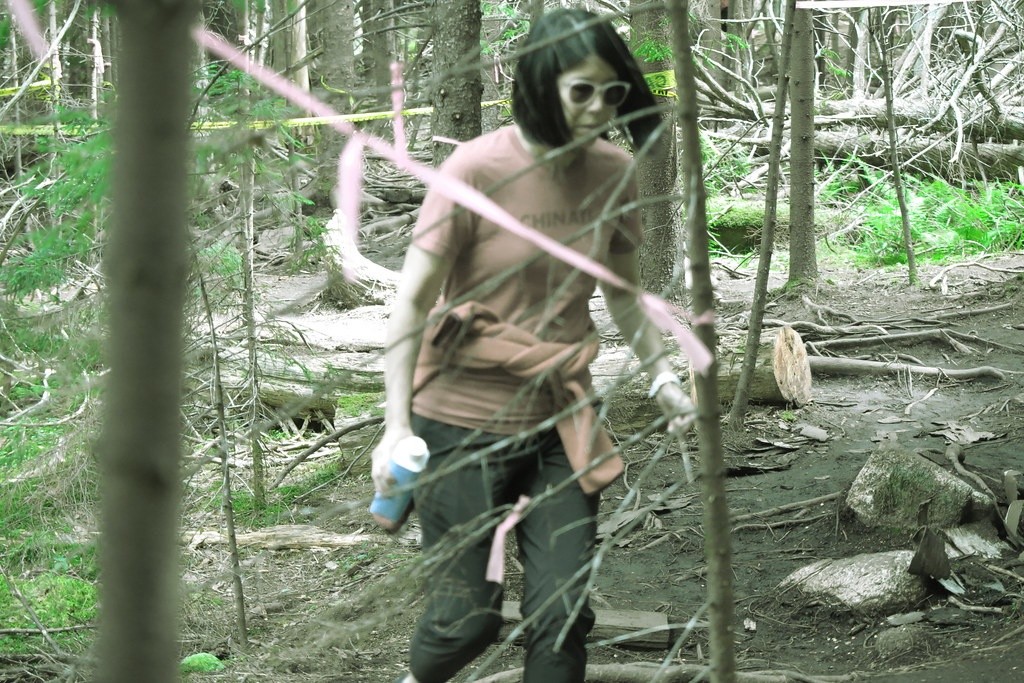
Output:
[370,9,696,682]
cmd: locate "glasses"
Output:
[559,76,631,109]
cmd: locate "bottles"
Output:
[370,436,431,533]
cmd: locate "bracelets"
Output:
[648,372,680,398]
